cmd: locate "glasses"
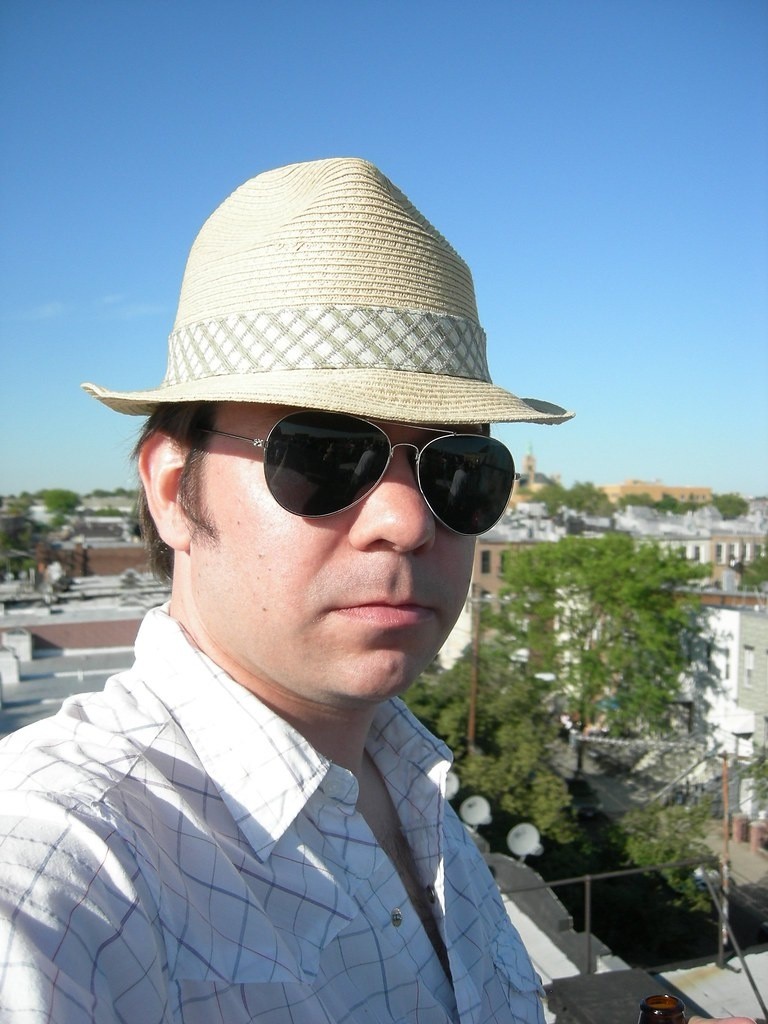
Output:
[195,409,521,536]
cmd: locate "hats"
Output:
[80,157,576,426]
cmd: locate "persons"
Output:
[0,157,757,1024]
